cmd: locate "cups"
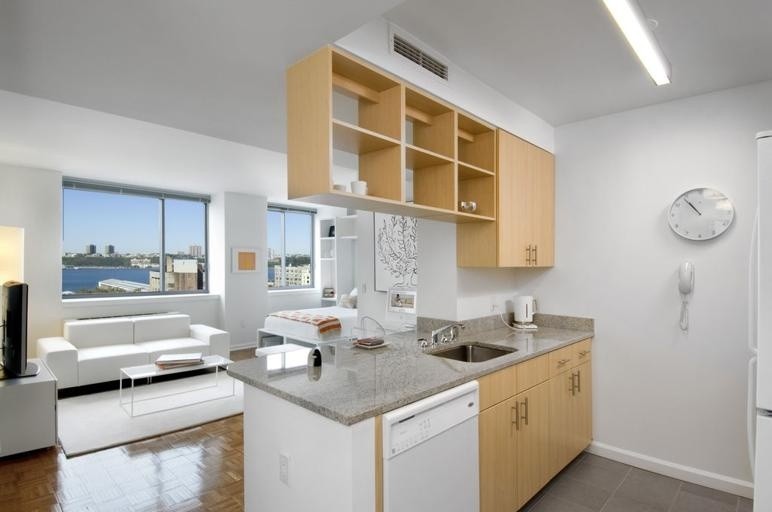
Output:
[350,180,368,196]
[334,185,346,192]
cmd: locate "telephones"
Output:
[679,261,694,294]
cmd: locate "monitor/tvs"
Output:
[0,281,29,377]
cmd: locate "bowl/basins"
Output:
[458,202,477,213]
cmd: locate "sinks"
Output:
[427,341,518,363]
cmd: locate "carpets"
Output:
[53,369,245,456]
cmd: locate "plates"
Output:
[354,341,391,349]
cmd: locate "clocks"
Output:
[669,186,733,241]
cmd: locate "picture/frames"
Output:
[230,246,261,275]
[384,290,417,316]
[372,212,417,292]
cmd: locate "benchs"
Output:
[255,342,302,358]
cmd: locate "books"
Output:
[155,353,203,364]
[159,362,203,368]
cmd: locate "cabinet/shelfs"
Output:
[457,125,556,267]
[282,43,497,220]
[320,216,362,306]
[1,358,58,463]
[479,354,552,511]
[552,336,593,477]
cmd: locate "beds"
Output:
[257,307,360,349]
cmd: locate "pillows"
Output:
[339,292,359,307]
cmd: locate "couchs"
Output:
[39,312,231,390]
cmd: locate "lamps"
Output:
[599,1,673,89]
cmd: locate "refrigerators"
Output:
[745,130,771,511]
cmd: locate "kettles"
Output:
[512,295,539,329]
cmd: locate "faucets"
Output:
[430,320,466,346]
